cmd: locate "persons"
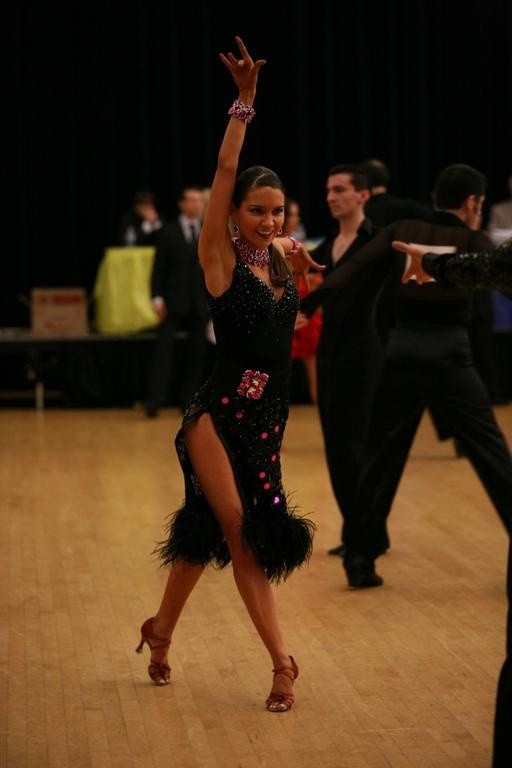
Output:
[300,164,512,590]
[307,164,391,558]
[133,32,328,714]
[392,238,512,768]
[124,184,388,421]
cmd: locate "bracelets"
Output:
[227,101,256,123]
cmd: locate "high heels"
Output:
[135,619,175,683]
[262,653,302,715]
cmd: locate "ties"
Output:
[189,224,200,245]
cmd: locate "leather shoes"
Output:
[342,554,384,588]
